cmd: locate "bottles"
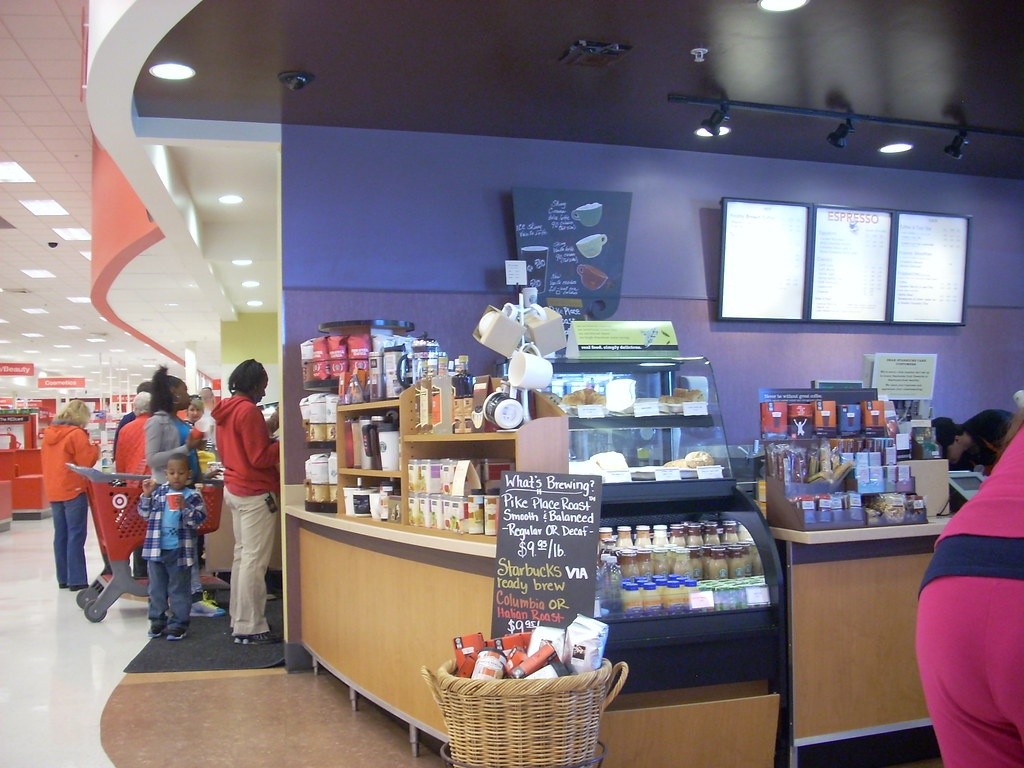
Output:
[415,353,473,438]
[484,487,500,536]
[594,519,768,614]
[467,488,484,533]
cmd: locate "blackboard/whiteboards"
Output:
[490,470,602,639]
[510,186,633,336]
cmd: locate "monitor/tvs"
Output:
[952,476,982,491]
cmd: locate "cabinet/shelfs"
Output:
[757,387,929,532]
[86,421,120,471]
[285,320,569,758]
[490,356,789,768]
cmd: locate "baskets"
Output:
[421,658,628,768]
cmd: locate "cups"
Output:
[478,303,518,337]
[342,486,383,520]
[521,246,548,293]
[192,420,210,438]
[166,492,183,511]
[576,264,608,290]
[571,204,602,228]
[483,382,523,429]
[523,286,538,308]
[576,234,608,258]
[508,344,554,390]
[524,304,546,340]
[345,413,399,471]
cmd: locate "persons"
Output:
[211,359,281,646]
[137,453,208,643]
[915,388,1024,768]
[931,408,1018,472]
[39,398,102,591]
[145,367,225,618]
[113,380,220,463]
[114,391,151,579]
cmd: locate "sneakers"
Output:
[166,628,186,639]
[243,633,282,645]
[148,625,167,636]
[234,635,243,643]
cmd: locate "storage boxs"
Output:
[451,632,569,679]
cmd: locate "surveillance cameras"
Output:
[278,72,314,91]
[48,243,58,248]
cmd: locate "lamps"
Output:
[942,130,969,161]
[826,118,855,149]
[278,70,316,90]
[700,104,730,136]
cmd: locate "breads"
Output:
[561,388,606,406]
[659,388,706,404]
[664,452,715,468]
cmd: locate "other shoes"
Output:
[70,583,89,591]
[59,583,69,588]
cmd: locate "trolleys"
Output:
[61,461,231,623]
